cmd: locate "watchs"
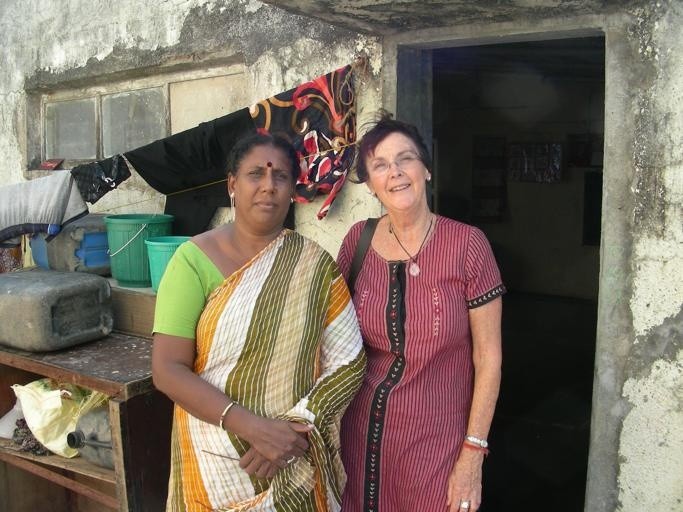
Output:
[463,434,493,448]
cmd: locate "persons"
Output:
[144,128,370,512]
[337,116,508,512]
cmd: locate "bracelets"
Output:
[218,400,239,432]
[460,441,491,455]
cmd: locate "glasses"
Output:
[372,150,421,174]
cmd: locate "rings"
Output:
[285,454,298,466]
[458,499,471,510]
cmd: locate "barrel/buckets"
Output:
[66,404,116,471]
[27,212,113,277]
[0,266,115,353]
[142,235,195,294]
[102,212,176,288]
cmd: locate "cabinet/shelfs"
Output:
[1,328,176,512]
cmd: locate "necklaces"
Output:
[386,215,436,279]
